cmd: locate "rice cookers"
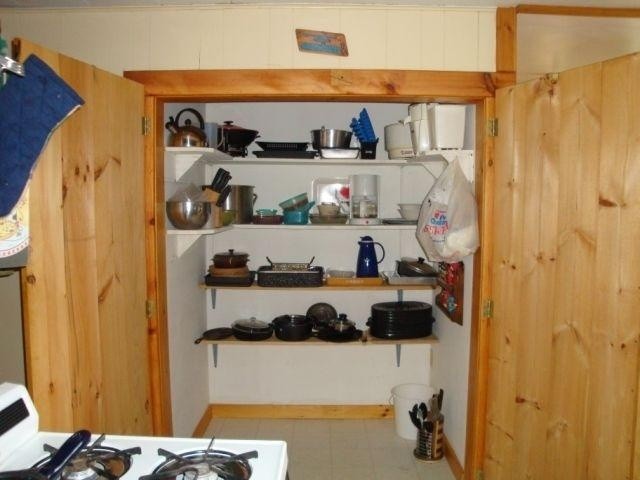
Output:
[384,122,412,159]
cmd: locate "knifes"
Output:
[212,168,233,207]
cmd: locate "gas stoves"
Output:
[1,432,289,480]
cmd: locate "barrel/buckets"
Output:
[388,382,436,440]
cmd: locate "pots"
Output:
[218,121,259,148]
[394,256,436,277]
[195,314,362,345]
[202,185,257,224]
[310,127,352,150]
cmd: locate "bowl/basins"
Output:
[397,203,421,220]
[166,201,211,229]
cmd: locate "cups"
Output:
[200,122,224,149]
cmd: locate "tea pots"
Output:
[356,235,385,277]
[165,109,210,148]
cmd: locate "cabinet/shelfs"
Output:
[6,37,640,480]
